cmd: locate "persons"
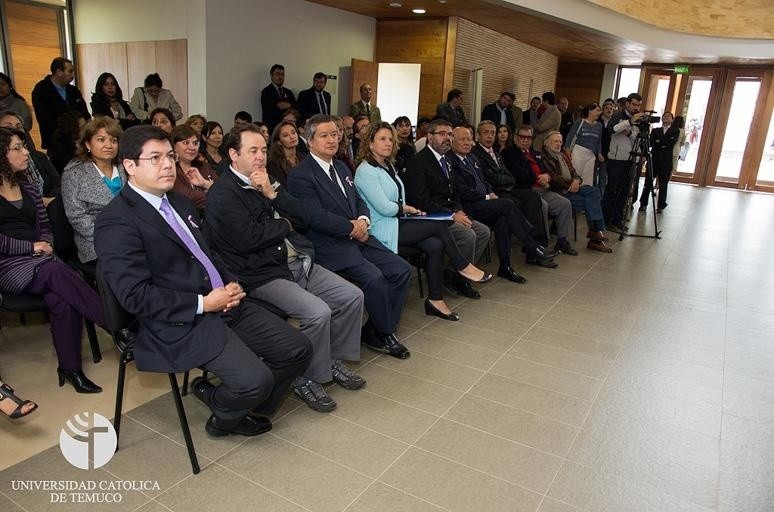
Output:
[3,58,696,438]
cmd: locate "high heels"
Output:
[424,297,460,321]
[57,363,102,394]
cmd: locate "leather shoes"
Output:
[520,238,578,270]
[453,259,494,300]
[497,264,527,284]
[587,228,613,253]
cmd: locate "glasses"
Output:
[138,152,179,166]
[434,131,453,137]
[518,134,533,141]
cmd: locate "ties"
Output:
[440,157,448,178]
[278,87,283,96]
[329,164,344,194]
[463,158,486,195]
[366,103,371,122]
[159,197,225,292]
[319,93,326,115]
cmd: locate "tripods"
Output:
[608,130,662,241]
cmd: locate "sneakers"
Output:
[604,222,629,232]
[361,323,411,360]
[324,356,367,391]
[292,372,338,414]
[190,375,274,438]
[639,204,664,214]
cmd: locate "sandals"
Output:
[0,375,39,420]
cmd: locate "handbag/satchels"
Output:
[562,118,585,154]
